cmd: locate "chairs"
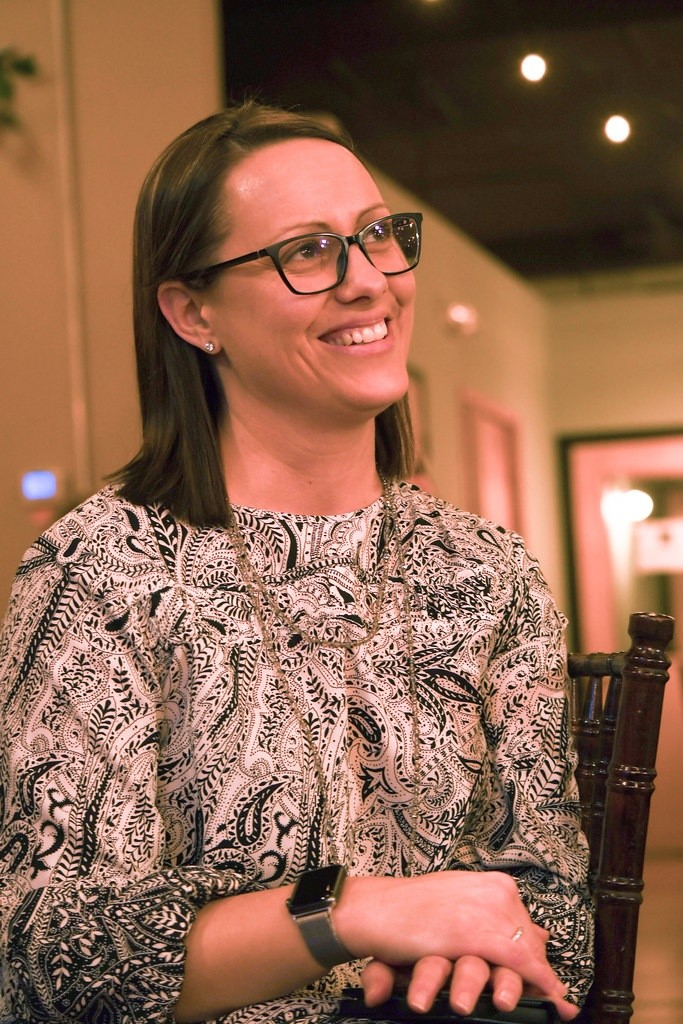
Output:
[568,612,677,1024]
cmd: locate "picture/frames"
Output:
[459,384,525,537]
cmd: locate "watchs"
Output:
[282,861,360,973]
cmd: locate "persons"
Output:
[0,101,596,1024]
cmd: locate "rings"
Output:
[512,929,523,942]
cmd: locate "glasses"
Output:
[177,211,425,296]
